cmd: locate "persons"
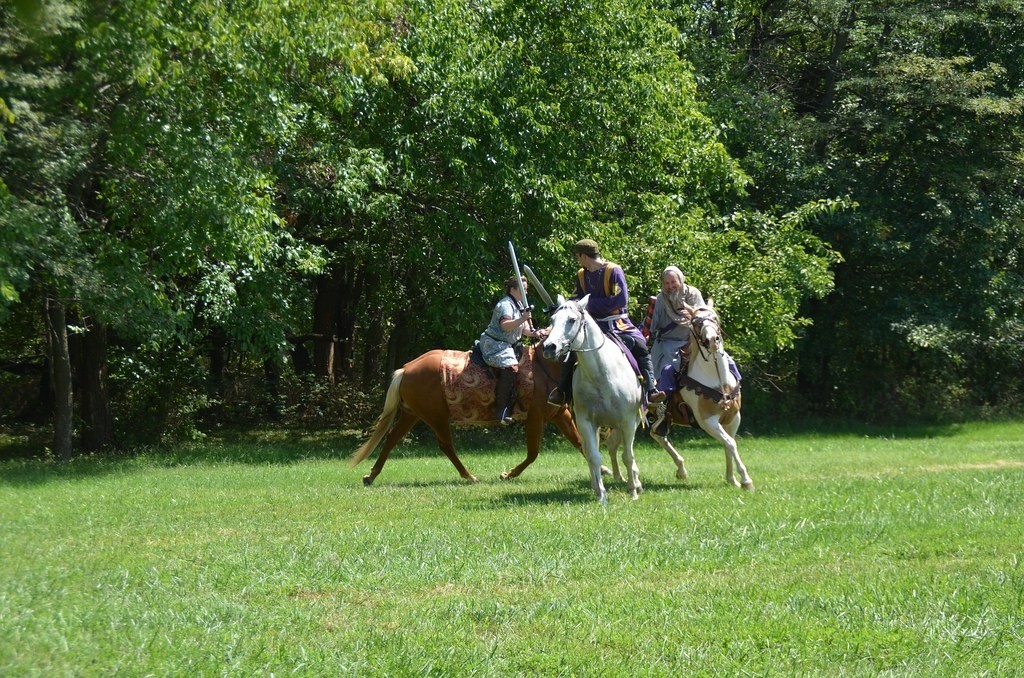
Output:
[480,275,548,424]
[647,266,742,435]
[547,240,666,406]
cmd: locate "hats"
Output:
[571,239,599,255]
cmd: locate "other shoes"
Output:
[648,389,666,403]
[547,386,572,407]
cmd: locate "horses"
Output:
[643,297,757,492]
[347,325,614,488]
[543,291,651,507]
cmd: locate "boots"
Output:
[492,370,518,421]
[664,390,674,418]
